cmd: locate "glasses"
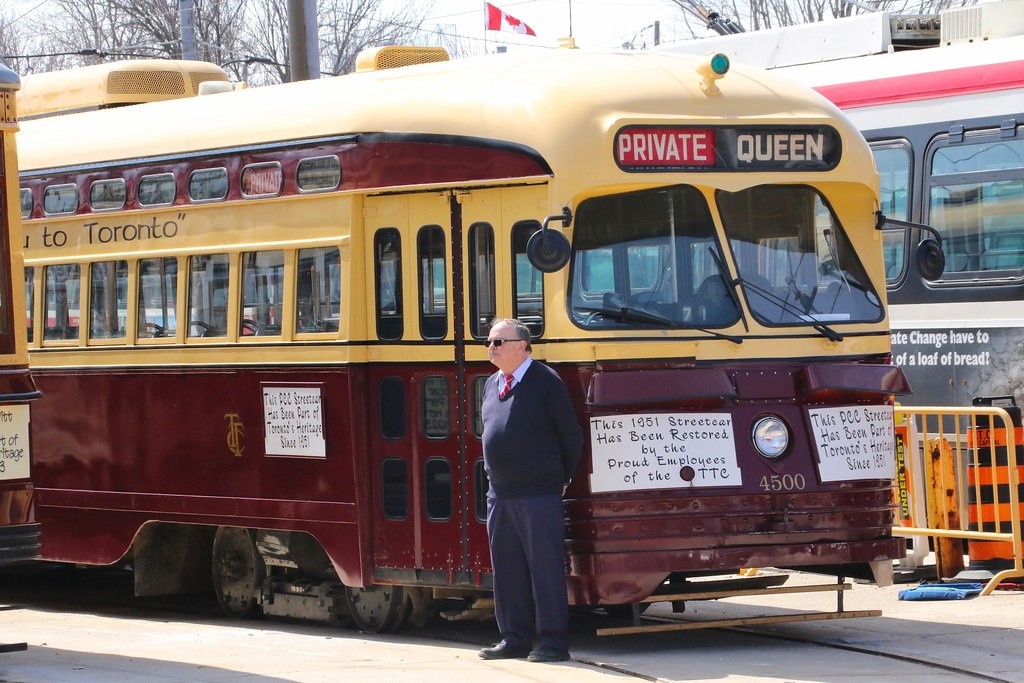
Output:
[484,340,521,347]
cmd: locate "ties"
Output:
[499,375,514,399]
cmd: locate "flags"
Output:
[485,2,537,37]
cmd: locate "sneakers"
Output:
[478,638,534,659]
[527,651,570,662]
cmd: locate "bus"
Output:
[639,0,1024,547]
[1,42,944,647]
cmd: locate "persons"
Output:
[479,318,585,664]
